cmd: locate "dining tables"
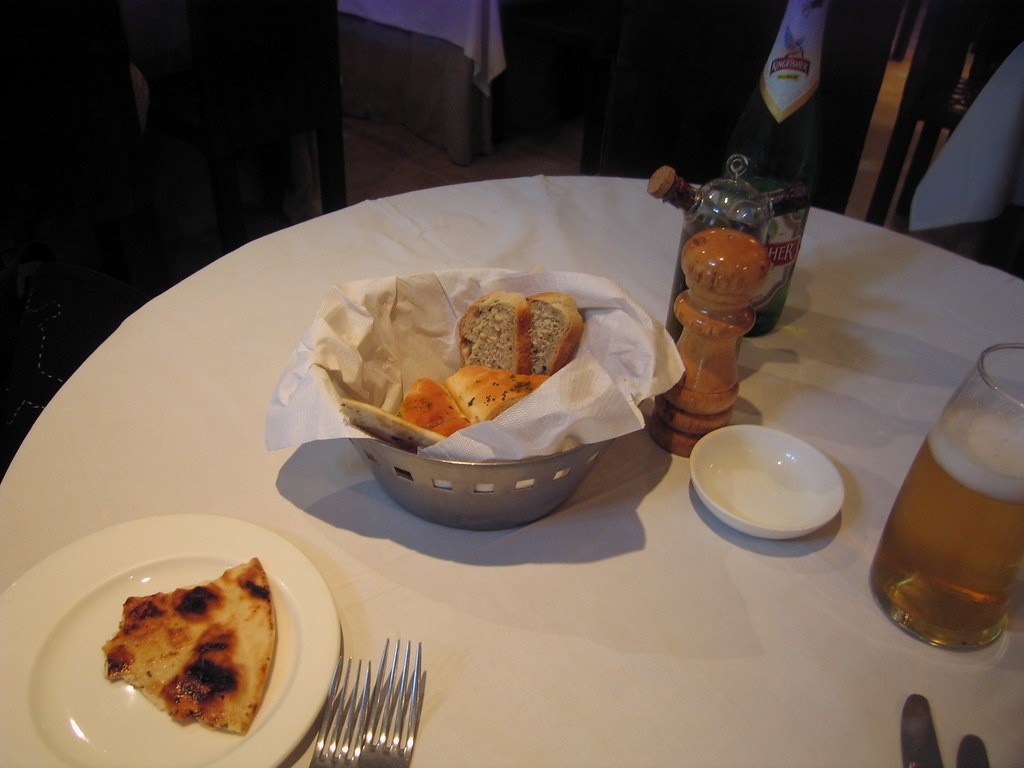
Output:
[1,176,1024,768]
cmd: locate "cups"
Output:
[869,342,1024,652]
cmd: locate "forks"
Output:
[310,637,422,768]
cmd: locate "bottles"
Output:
[664,0,830,341]
[646,155,810,457]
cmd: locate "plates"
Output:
[0,513,343,768]
[690,425,845,540]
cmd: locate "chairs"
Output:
[837,0,1024,277]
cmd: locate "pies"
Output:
[102,557,277,734]
[339,399,447,453]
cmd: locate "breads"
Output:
[395,290,583,438]
